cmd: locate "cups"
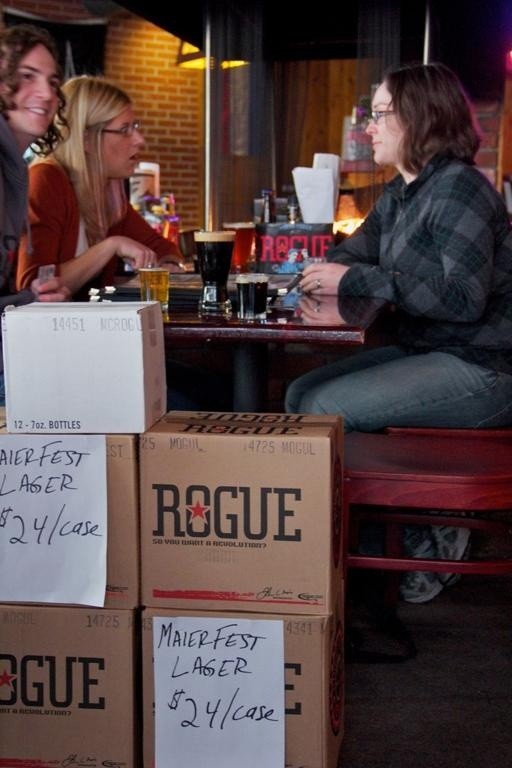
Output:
[138,267,170,313]
[236,273,269,321]
[194,222,256,314]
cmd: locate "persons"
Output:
[0,20,74,416]
[278,59,511,607]
[292,290,390,326]
[2,72,233,414]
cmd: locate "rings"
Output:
[313,278,320,285]
[147,263,156,269]
[313,299,323,313]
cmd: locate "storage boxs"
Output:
[2,608,138,765]
[0,435,142,610]
[136,411,343,618]
[137,609,347,765]
[2,301,170,434]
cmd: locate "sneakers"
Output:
[398,522,473,605]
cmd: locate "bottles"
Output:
[259,188,278,222]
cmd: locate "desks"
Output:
[82,287,382,408]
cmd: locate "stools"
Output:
[336,414,505,586]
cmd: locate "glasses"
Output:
[370,109,399,123]
[89,118,141,137]
